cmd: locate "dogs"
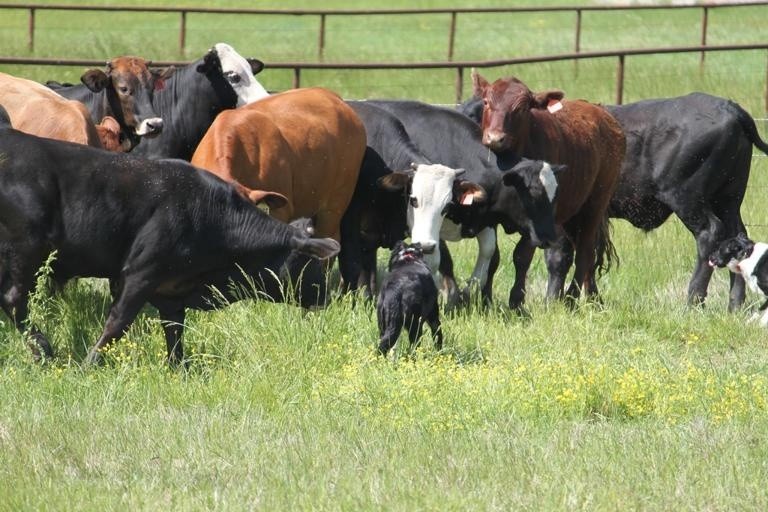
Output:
[708,232,768,327]
[375,240,443,358]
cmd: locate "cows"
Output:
[457,92,768,317]
[352,98,570,318]
[0,42,270,164]
[187,85,367,271]
[0,127,342,378]
[471,66,628,319]
[340,96,467,318]
